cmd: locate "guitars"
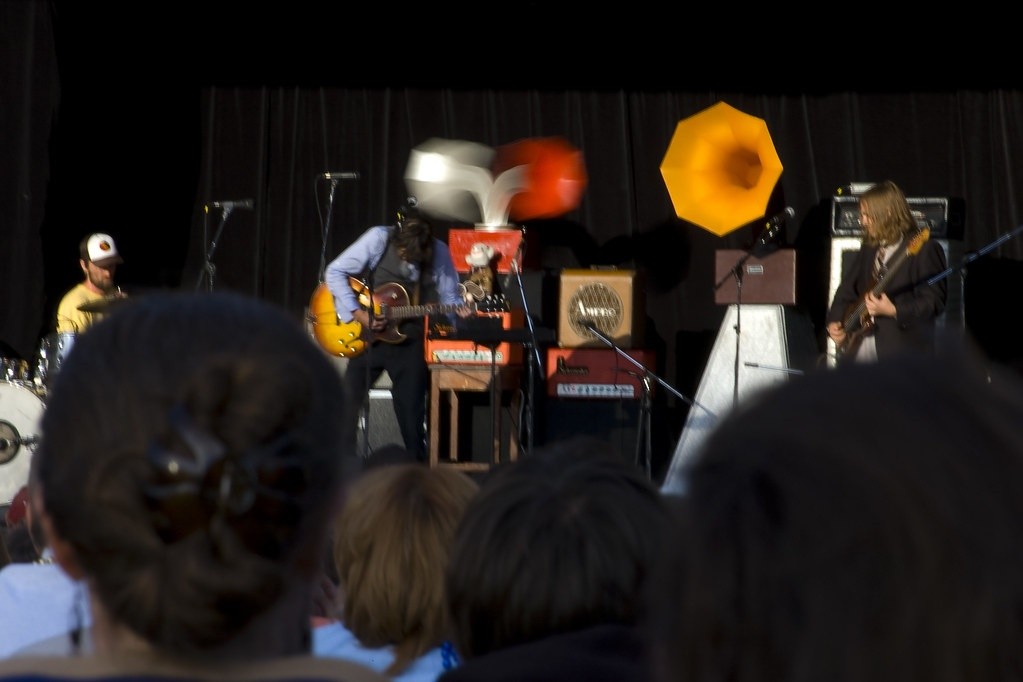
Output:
[309,274,512,358]
[835,226,930,369]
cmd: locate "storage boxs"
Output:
[714,248,797,304]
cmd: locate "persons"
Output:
[0,293,379,682]
[439,356,1023,682]
[334,461,478,682]
[324,205,479,463]
[58,232,130,360]
[825,182,947,362]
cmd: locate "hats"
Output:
[79,234,121,266]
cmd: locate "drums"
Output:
[32,332,83,398]
[0,358,30,388]
[0,378,49,507]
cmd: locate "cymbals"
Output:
[75,296,129,313]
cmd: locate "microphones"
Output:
[767,206,796,225]
[578,320,596,330]
[209,198,254,209]
[317,171,360,182]
[397,195,418,216]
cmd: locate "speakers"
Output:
[353,388,429,461]
[557,269,641,350]
[715,247,799,307]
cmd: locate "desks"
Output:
[429,364,517,471]
